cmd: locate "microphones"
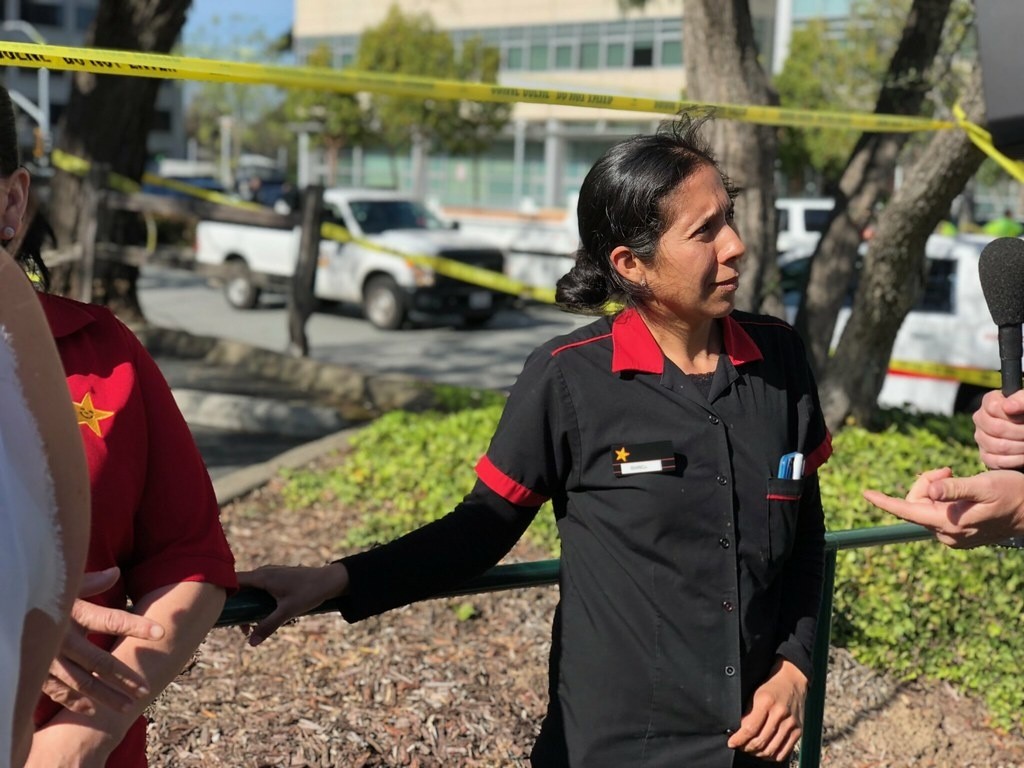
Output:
[978,237,1024,397]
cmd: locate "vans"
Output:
[775,197,834,254]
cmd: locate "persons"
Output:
[228,104,834,768]
[937,198,1024,236]
[863,388,1024,549]
[1,85,239,768]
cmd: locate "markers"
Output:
[791,453,803,480]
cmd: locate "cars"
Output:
[777,235,1004,422]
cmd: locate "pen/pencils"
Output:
[785,457,794,479]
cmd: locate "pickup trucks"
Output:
[190,186,508,331]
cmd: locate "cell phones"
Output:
[777,452,798,479]
